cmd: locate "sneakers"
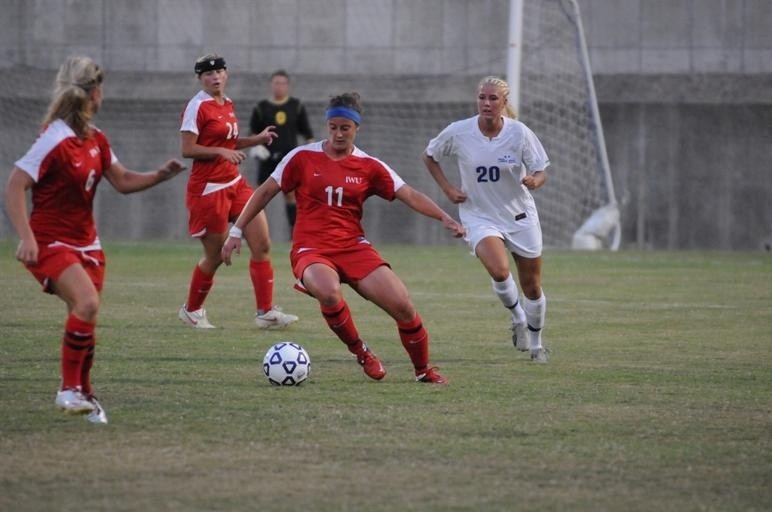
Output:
[530,347,553,362]
[254,305,300,330]
[55,384,108,425]
[510,310,530,352]
[414,364,449,384]
[179,303,216,329]
[356,346,386,380]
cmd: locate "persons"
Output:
[424,76,550,362]
[221,92,467,384]
[177,54,299,330]
[249,70,315,240]
[4,56,188,422]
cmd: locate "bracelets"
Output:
[229,226,242,238]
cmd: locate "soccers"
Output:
[263,341,311,387]
